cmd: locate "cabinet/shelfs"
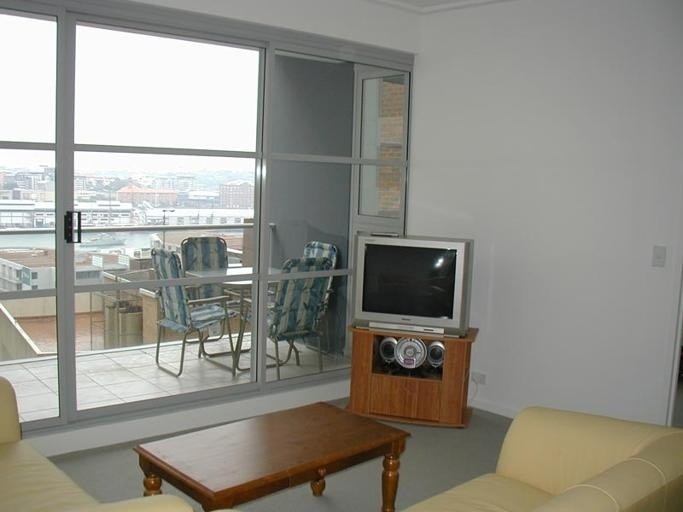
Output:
[343,320,478,429]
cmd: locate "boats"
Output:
[79,232,126,246]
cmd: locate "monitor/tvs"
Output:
[353,233,474,338]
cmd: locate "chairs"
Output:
[150,235,338,381]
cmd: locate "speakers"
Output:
[370,335,444,379]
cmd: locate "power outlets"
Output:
[469,370,484,384]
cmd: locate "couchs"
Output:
[1,375,241,512]
[397,406,682,512]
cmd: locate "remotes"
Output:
[371,231,399,238]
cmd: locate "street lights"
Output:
[161,208,176,248]
